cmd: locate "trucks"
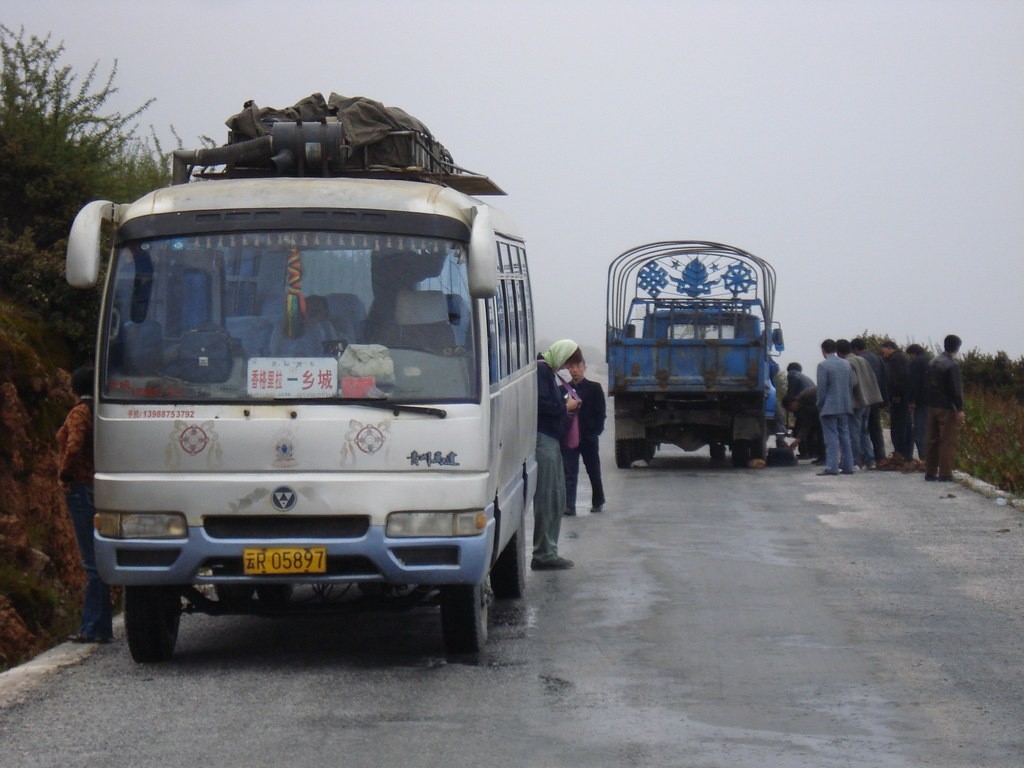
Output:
[603,239,785,469]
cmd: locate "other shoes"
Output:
[925,474,937,481]
[840,470,856,474]
[817,469,839,476]
[564,508,576,515]
[531,557,574,570]
[67,632,105,642]
[591,504,603,512]
[939,476,952,481]
[884,452,904,470]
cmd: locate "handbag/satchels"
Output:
[339,344,396,385]
[178,323,232,383]
[767,448,794,466]
[564,413,580,449]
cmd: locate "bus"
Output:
[62,118,542,665]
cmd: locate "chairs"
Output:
[224,287,471,358]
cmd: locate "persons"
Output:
[306,249,446,345]
[529,343,607,570]
[54,366,113,643]
[768,335,966,482]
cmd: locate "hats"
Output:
[72,367,94,390]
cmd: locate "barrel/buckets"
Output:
[342,377,376,398]
